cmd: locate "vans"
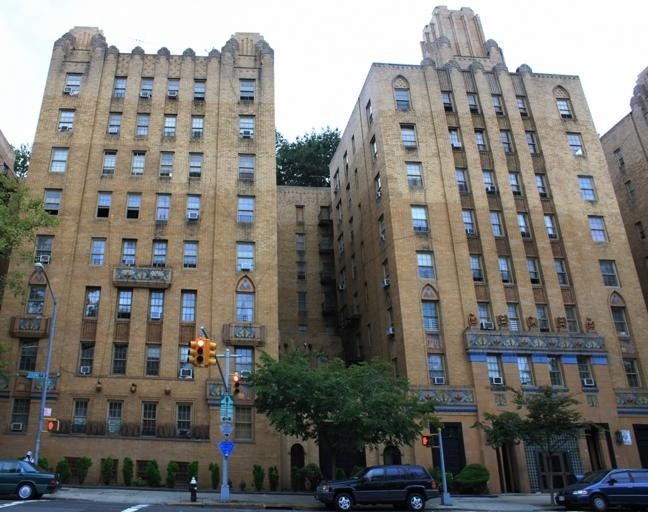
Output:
[551,467,648,512]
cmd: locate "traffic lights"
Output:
[206,339,218,367]
[47,420,59,431]
[231,374,241,396]
[186,336,197,366]
[422,434,433,447]
[194,336,208,368]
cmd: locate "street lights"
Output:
[27,258,61,469]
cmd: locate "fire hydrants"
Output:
[189,476,199,502]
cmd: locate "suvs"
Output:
[315,461,438,511]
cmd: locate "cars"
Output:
[0,459,63,499]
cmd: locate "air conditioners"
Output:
[39,254,51,262]
[168,90,179,97]
[241,370,251,379]
[140,91,150,97]
[63,87,72,93]
[150,311,161,319]
[466,228,475,234]
[241,263,250,270]
[434,375,446,385]
[188,211,198,219]
[10,422,23,430]
[60,125,69,132]
[109,127,118,134]
[180,368,191,376]
[80,365,91,373]
[482,321,494,330]
[584,378,596,386]
[243,130,253,137]
[486,186,496,192]
[125,258,135,265]
[489,376,503,385]
[381,279,388,287]
[385,326,394,335]
